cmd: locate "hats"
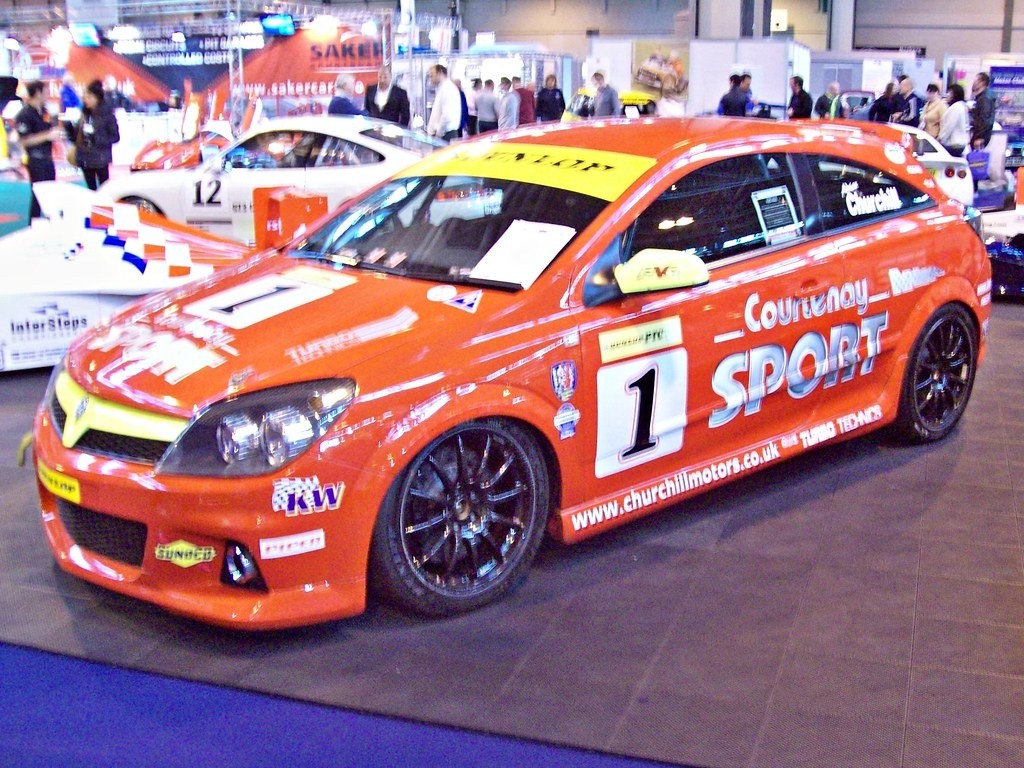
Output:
[87,80,104,100]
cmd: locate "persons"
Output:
[101,75,132,114]
[365,65,410,161]
[717,74,758,118]
[59,72,84,144]
[16,79,64,225]
[786,76,813,120]
[815,82,845,120]
[738,75,758,117]
[466,72,622,136]
[426,64,462,151]
[851,72,997,158]
[0,76,26,164]
[328,74,370,165]
[58,80,121,192]
[452,79,469,138]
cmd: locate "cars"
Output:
[33,119,992,631]
[0,118,504,372]
[883,123,974,206]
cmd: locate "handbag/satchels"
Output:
[966,146,990,180]
[67,146,80,167]
